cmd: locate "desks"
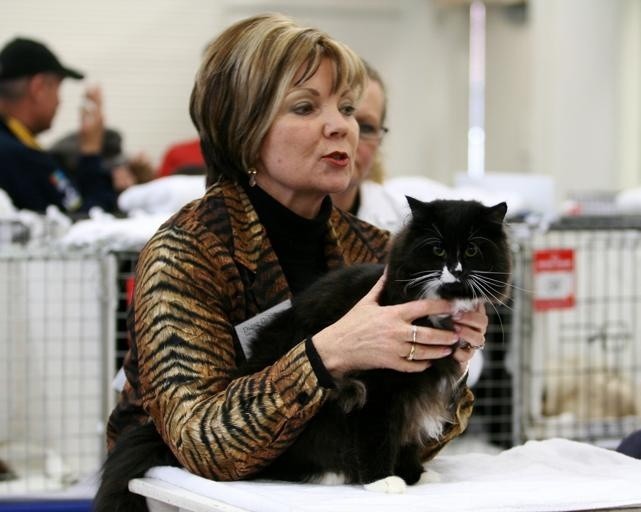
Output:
[127,478,247,512]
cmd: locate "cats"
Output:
[87,193,535,512]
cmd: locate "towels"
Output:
[145,436,641,511]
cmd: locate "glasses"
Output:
[358,123,388,139]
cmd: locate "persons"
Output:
[339,55,412,233]
[49,86,122,161]
[0,37,135,218]
[92,14,489,512]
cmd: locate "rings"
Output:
[412,324,418,343]
[407,344,416,361]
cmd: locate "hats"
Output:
[0,38,83,81]
